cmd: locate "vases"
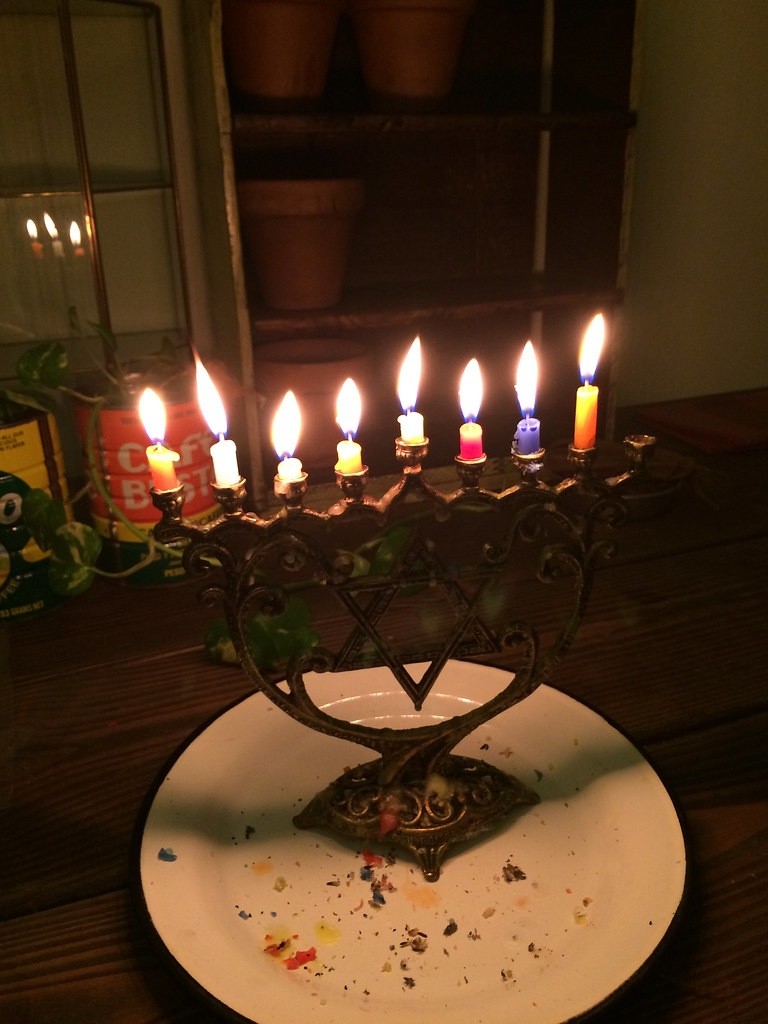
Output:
[229,0,338,103]
[357,0,476,112]
[253,338,372,470]
[240,177,365,311]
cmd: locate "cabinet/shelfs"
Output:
[182,0,647,506]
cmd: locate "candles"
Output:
[394,336,426,442]
[334,375,363,473]
[24,218,43,258]
[191,348,238,486]
[271,391,305,482]
[456,357,486,460]
[515,337,542,455]
[69,220,84,256]
[136,385,180,492]
[45,210,65,258]
[574,314,607,449]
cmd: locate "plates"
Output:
[140,658,688,1024]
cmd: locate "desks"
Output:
[0,390,768,1024]
